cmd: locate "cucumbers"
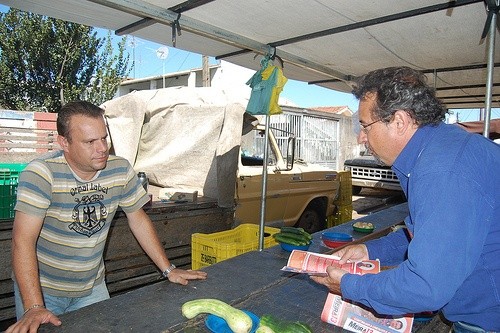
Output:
[272,226,313,246]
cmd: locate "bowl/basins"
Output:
[205,310,260,333]
[352,224,375,233]
[322,232,352,241]
[280,242,312,251]
[320,237,354,249]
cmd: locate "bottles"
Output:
[138,172,147,193]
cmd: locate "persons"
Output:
[3,100,207,333]
[310,67,500,333]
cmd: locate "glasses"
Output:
[359,109,412,134]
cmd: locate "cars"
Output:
[344,149,407,198]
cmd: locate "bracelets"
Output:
[24,304,45,316]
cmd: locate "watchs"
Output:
[162,264,176,277]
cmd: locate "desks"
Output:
[27,202,454,333]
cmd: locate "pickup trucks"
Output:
[0,85,342,322]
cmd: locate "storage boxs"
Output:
[191,223,281,270]
[0,162,30,221]
